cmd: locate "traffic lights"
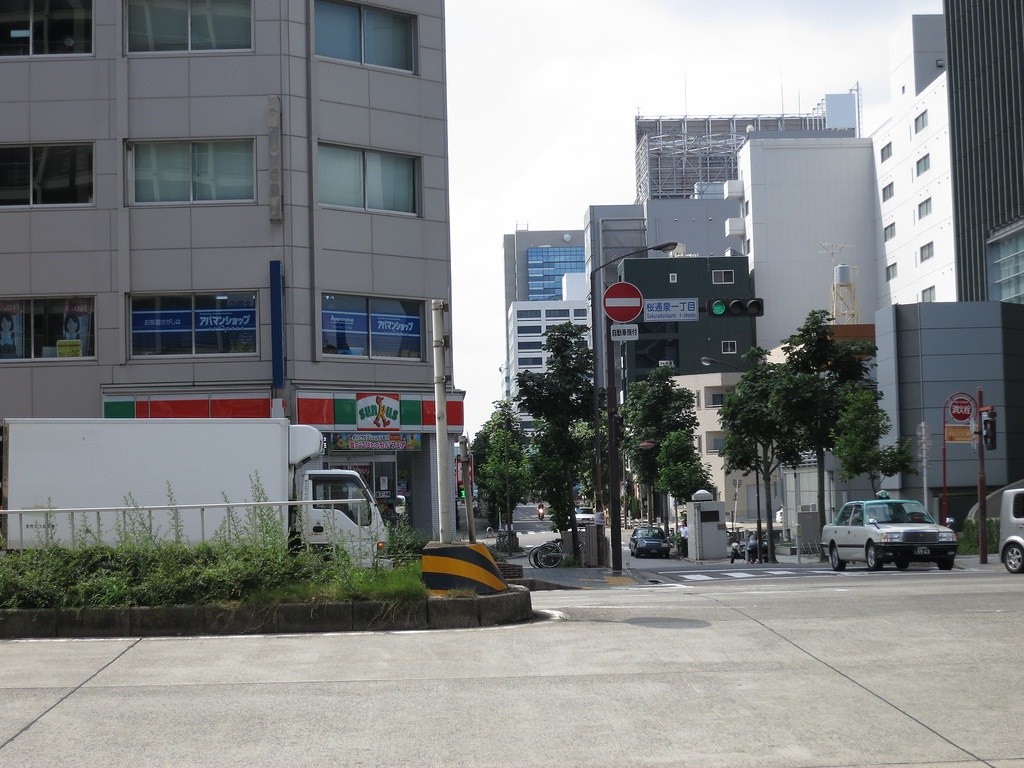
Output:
[709,299,761,318]
[983,419,996,450]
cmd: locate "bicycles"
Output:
[496,529,517,552]
[527,538,585,569]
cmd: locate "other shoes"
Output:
[682,556,687,558]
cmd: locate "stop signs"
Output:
[602,282,645,323]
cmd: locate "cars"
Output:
[998,489,1024,574]
[819,490,959,571]
[775,505,783,523]
[575,506,595,527]
[629,526,670,559]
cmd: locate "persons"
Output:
[677,521,688,558]
[870,508,877,523]
[536,502,544,519]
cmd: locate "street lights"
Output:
[590,241,678,570]
[701,357,762,565]
[624,442,655,530]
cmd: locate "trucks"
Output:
[3,417,395,572]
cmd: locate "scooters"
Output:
[536,508,545,521]
[747,531,768,564]
[727,530,746,564]
